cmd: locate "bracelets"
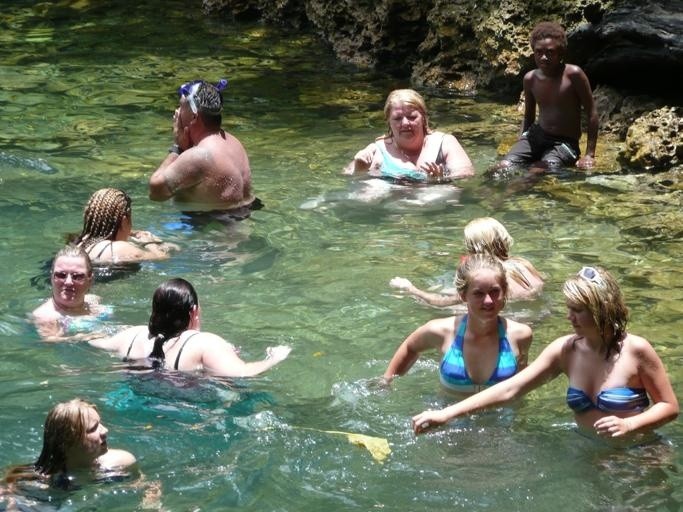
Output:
[143,239,159,247]
[168,146,184,155]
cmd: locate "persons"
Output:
[411,266,680,438]
[0,398,138,512]
[380,255,533,394]
[149,82,253,210]
[70,187,180,280]
[87,278,292,377]
[478,21,599,211]
[343,88,473,180]
[390,217,544,307]
[32,245,114,342]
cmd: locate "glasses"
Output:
[53,271,88,280]
[180,79,204,117]
[576,265,604,287]
